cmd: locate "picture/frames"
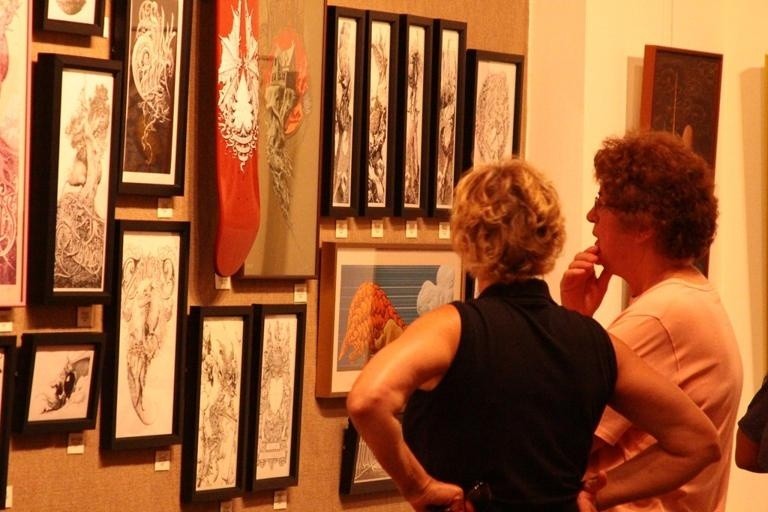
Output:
[35,53,123,304]
[463,49,522,177]
[319,6,361,219]
[397,14,436,219]
[111,0,193,196]
[425,18,468,221]
[355,10,400,217]
[623,45,724,314]
[0,331,17,509]
[17,332,103,434]
[339,415,399,498]
[0,0,33,307]
[181,306,254,503]
[36,0,105,37]
[238,1,326,281]
[101,220,190,452]
[315,239,476,399]
[246,303,307,493]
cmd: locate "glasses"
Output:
[594,195,623,211]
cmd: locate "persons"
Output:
[734,370,767,474]
[346,159,724,512]
[559,128,744,512]
